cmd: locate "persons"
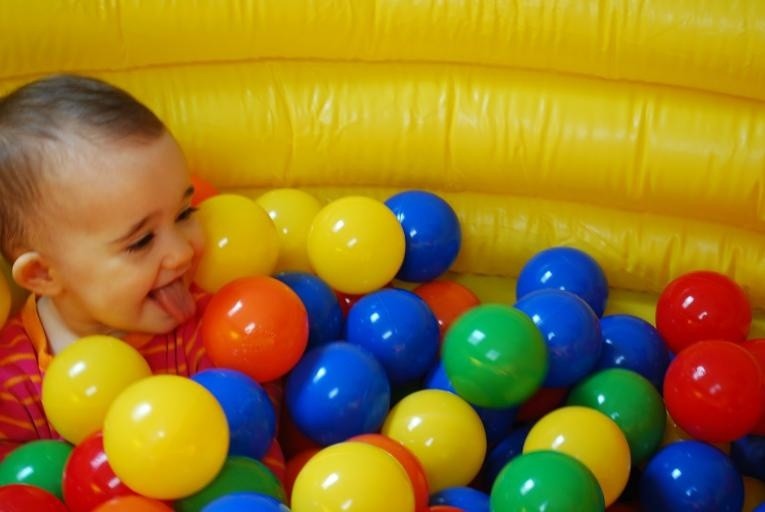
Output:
[0,74,290,491]
